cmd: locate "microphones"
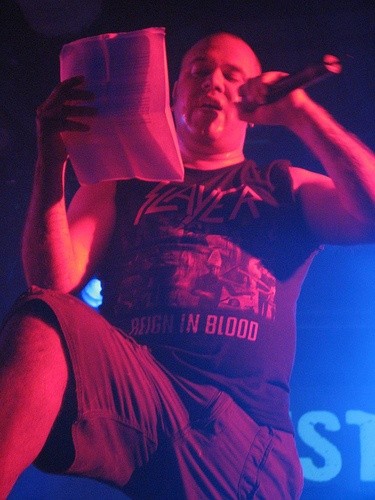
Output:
[241,54,341,111]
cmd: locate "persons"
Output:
[0,31,375,500]
